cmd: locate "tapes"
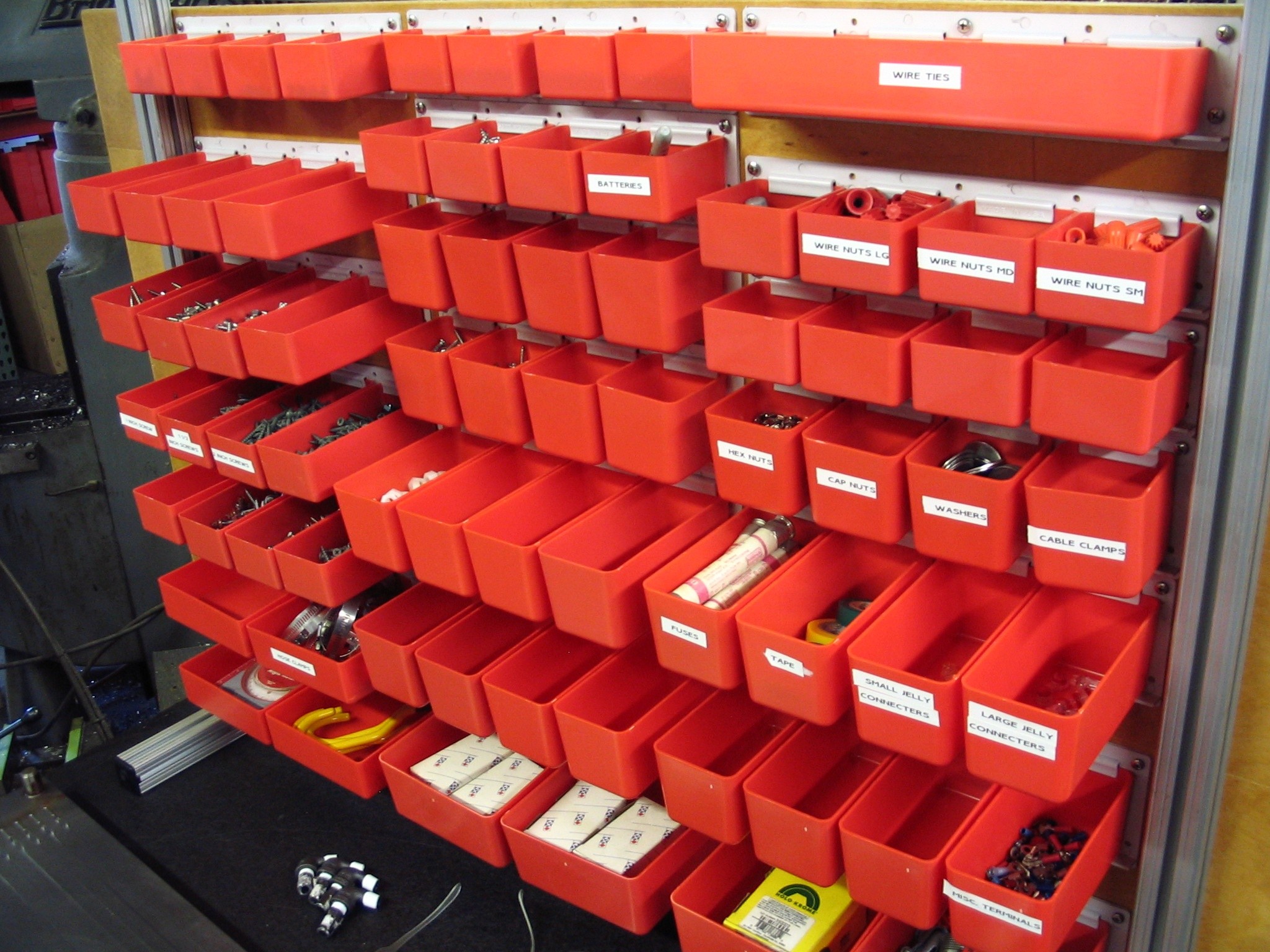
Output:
[804,599,875,645]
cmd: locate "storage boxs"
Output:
[0,32,1210,952]
